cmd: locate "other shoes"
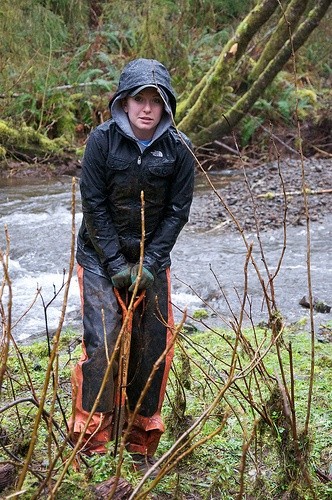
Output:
[81,453,165,479]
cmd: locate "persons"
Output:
[67,58,195,479]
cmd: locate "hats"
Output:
[126,84,169,103]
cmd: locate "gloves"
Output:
[110,264,155,292]
